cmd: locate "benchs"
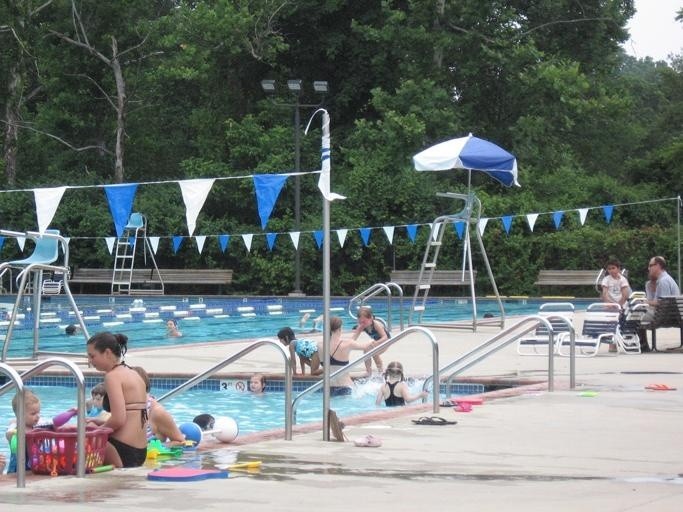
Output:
[68,267,233,295]
[534,268,605,294]
[386,269,477,297]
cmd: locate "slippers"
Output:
[352,432,382,448]
[439,394,482,412]
[328,408,346,443]
[410,413,459,429]
[644,382,677,392]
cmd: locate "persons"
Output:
[250,305,428,408]
[631,255,681,353]
[602,260,632,353]
[66,324,80,336]
[166,320,182,337]
[0,332,221,476]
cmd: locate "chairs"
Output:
[517,292,683,357]
[23,265,72,295]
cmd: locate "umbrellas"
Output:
[413,132,523,217]
